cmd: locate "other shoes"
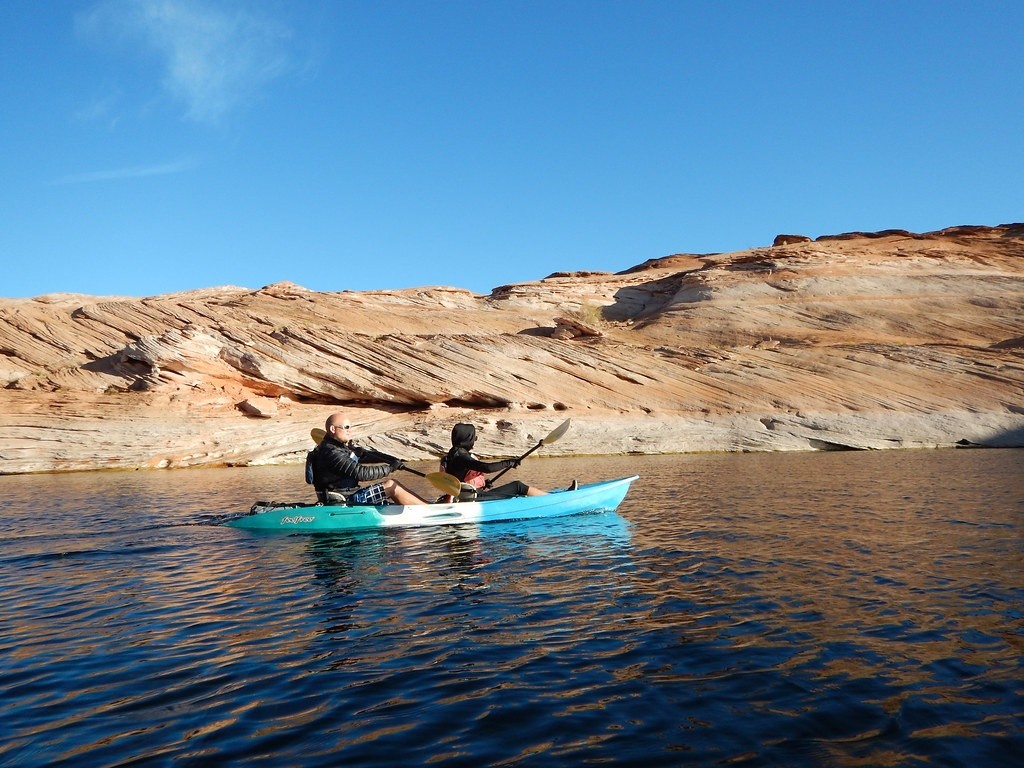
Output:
[435,494,454,503]
[567,480,577,491]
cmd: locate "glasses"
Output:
[475,436,478,441]
[335,425,352,430]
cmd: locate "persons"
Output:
[439,423,577,502]
[306,413,453,505]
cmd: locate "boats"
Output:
[226,473,643,531]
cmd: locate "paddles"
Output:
[309,428,461,497]
[483,417,571,490]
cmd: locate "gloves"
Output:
[388,459,404,473]
[510,456,521,468]
